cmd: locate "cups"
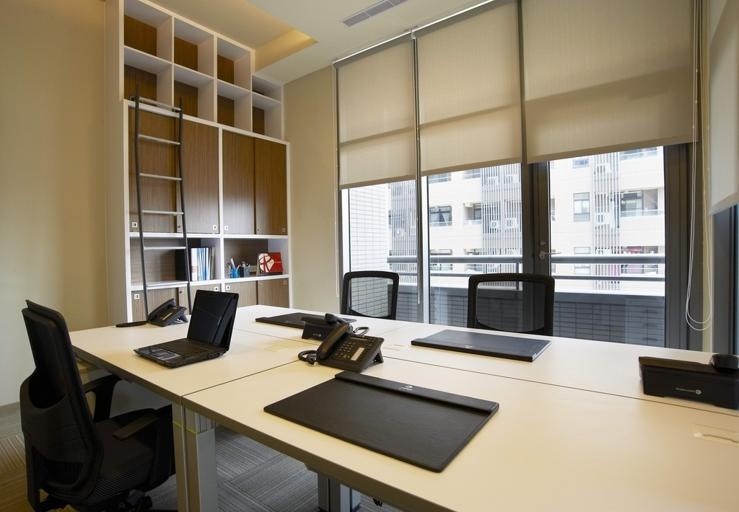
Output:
[280,224,286,235]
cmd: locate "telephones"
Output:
[301,314,353,340]
[298,324,384,372]
[147,299,187,327]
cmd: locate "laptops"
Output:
[133,290,239,368]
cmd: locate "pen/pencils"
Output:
[230,258,239,269]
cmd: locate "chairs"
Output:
[468,272,554,335]
[341,271,399,319]
[20,300,176,512]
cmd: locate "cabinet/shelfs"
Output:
[104,0,294,326]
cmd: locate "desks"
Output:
[233,304,405,344]
[70,313,330,511]
[183,355,739,512]
[372,322,739,418]
